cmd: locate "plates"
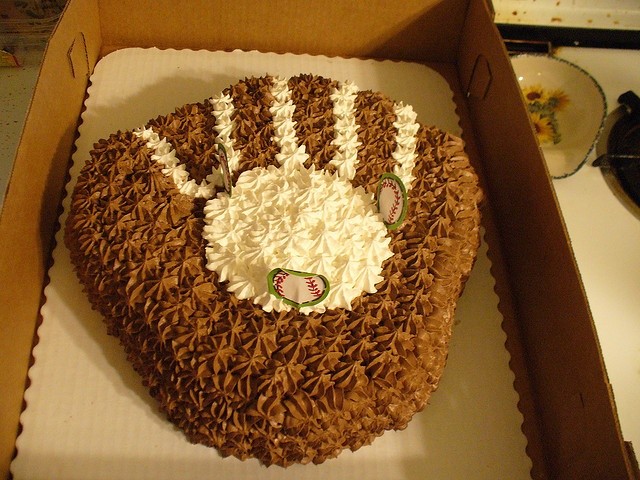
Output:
[506,51,608,183]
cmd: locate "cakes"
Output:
[65,73,483,468]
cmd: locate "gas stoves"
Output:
[504,35,640,477]
